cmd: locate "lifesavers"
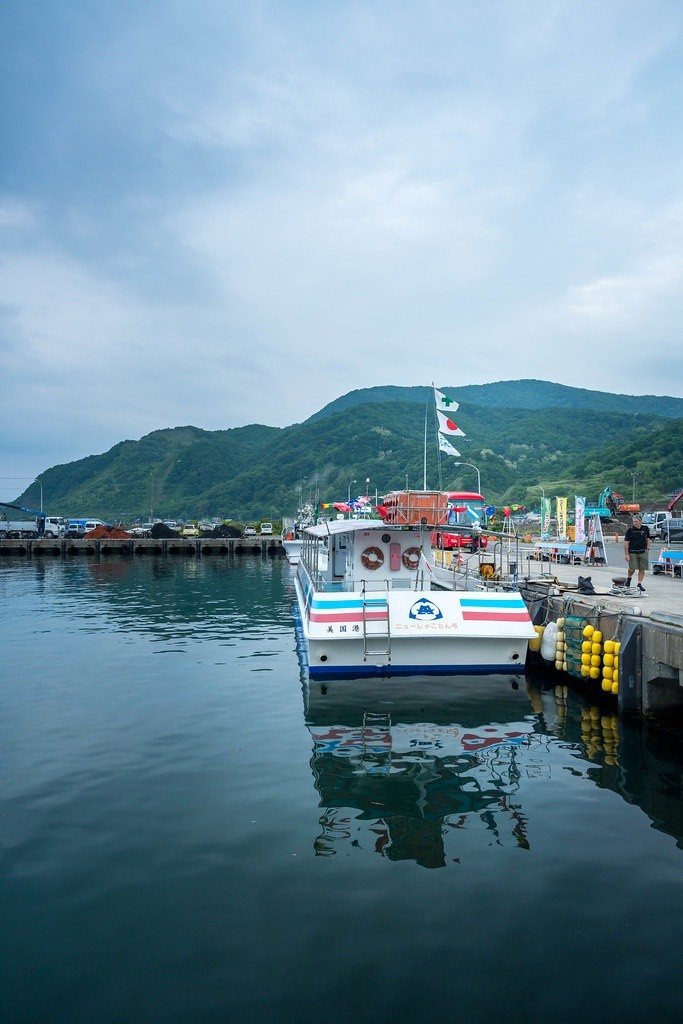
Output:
[361,547,385,571]
[402,547,421,569]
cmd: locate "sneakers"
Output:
[625,577,631,586]
[637,583,645,591]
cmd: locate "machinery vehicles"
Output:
[605,489,642,516]
[584,485,618,522]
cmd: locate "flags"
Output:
[347,500,362,509]
[452,508,469,513]
[485,507,495,517]
[419,550,440,582]
[355,496,371,508]
[376,505,388,517]
[435,389,459,413]
[332,502,351,512]
[447,508,455,517]
[466,506,482,520]
[437,432,462,457]
[502,507,512,518]
[436,410,466,437]
[511,504,526,513]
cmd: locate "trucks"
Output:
[642,511,683,539]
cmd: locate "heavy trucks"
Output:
[0,516,68,539]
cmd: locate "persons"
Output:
[471,523,486,554]
[623,514,650,591]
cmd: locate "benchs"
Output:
[650,551,683,578]
[431,577,466,591]
[534,542,586,566]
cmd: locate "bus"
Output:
[430,491,495,551]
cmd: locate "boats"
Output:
[280,489,540,712]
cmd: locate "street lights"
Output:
[454,462,481,495]
[34,479,43,512]
[348,480,357,519]
[366,478,370,497]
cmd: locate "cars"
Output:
[69,521,273,537]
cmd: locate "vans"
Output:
[660,518,683,543]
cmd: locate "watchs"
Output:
[647,547,649,550]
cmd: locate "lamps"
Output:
[421,517,427,524]
